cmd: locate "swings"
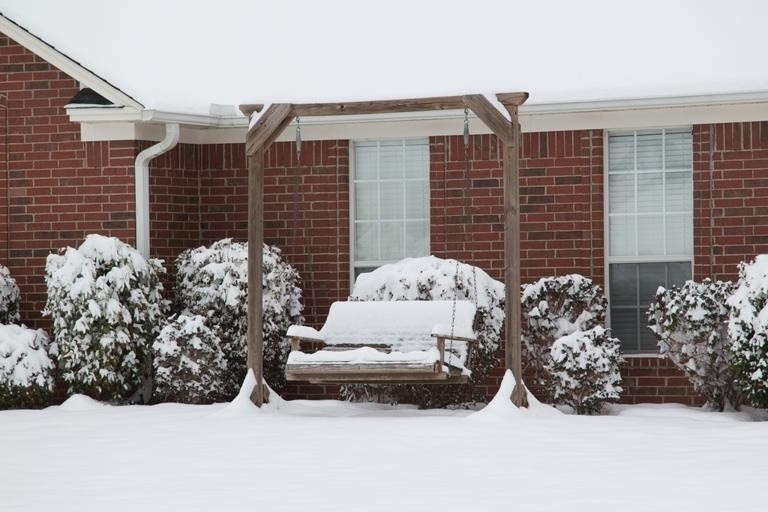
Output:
[284,108,478,385]
